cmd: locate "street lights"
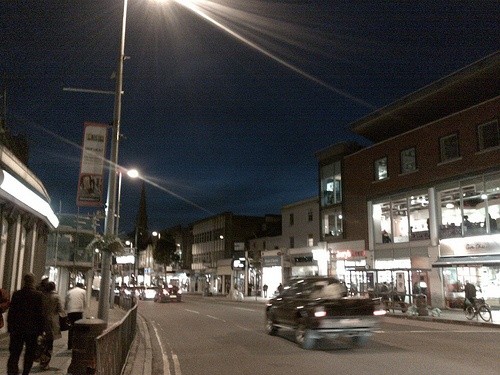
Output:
[110,167,139,309]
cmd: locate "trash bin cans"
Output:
[70,317,107,375]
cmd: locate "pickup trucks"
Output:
[265,276,375,350]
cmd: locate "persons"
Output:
[0,273,87,375]
[226,213,500,314]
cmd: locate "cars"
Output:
[112,286,181,302]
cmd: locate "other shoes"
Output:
[40,365,48,370]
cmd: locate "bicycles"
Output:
[464,296,491,322]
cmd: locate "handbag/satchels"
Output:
[59,311,72,332]
